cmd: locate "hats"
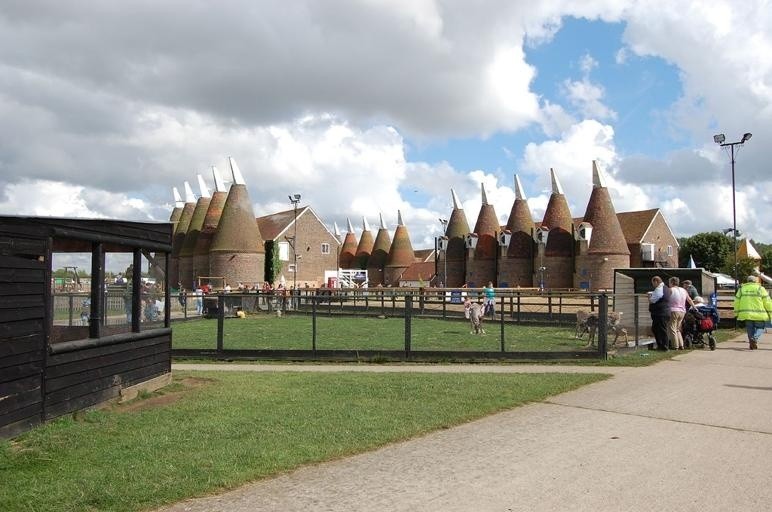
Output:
[692,296,704,303]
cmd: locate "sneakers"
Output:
[749,338,758,350]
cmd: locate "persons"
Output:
[223,284,231,294]
[141,280,164,323]
[377,282,392,288]
[664,275,699,350]
[123,293,132,327]
[646,274,671,351]
[732,274,771,351]
[687,294,708,310]
[195,281,212,315]
[464,281,496,334]
[179,288,187,312]
[239,281,286,295]
[290,282,315,289]
[682,279,703,344]
[81,291,90,325]
[439,282,443,288]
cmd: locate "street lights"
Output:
[289,194,301,288]
[439,218,448,288]
[715,132,753,331]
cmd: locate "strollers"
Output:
[683,304,719,351]
